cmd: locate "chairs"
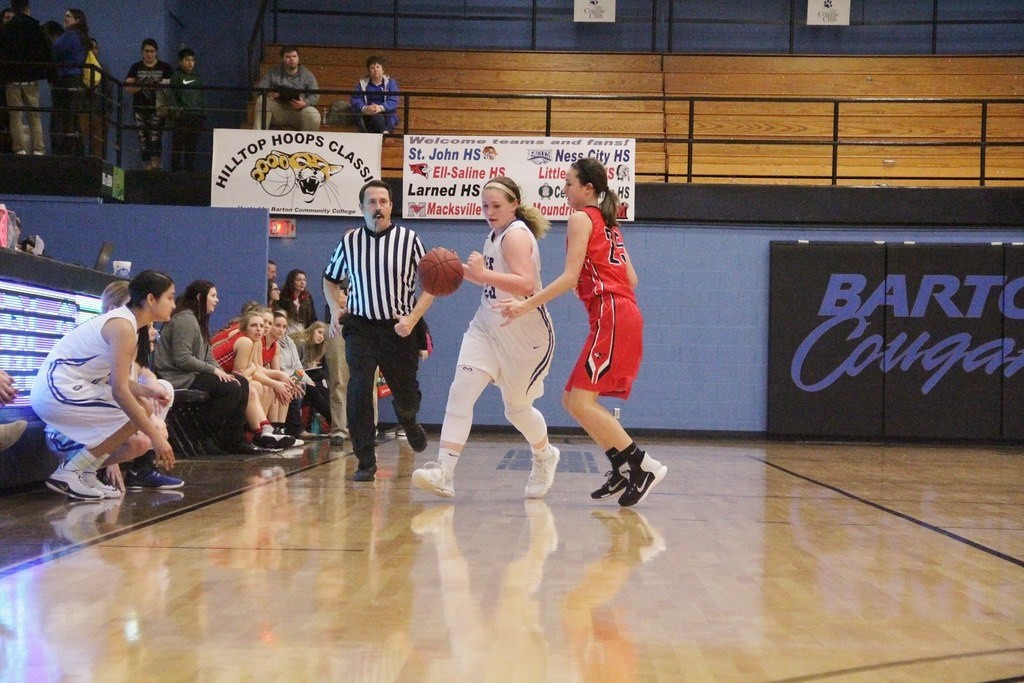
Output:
[165,389,222,457]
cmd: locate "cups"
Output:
[290,369,304,385]
[113,261,131,279]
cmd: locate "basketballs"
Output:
[417,250,464,296]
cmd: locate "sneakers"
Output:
[402,417,427,451]
[591,461,628,499]
[591,511,631,564]
[83,471,121,498]
[124,488,184,511]
[412,462,455,497]
[525,445,560,499]
[618,451,667,507]
[619,508,660,563]
[126,468,185,489]
[526,500,558,553]
[47,501,104,528]
[411,503,454,534]
[45,464,104,500]
[353,463,377,481]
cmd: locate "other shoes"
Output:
[330,436,344,446]
[252,443,284,452]
[16,150,26,154]
[396,429,406,436]
[260,427,296,447]
[33,149,43,155]
[384,426,397,435]
[298,430,318,440]
[0,420,27,452]
[292,438,305,446]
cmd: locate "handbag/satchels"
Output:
[327,101,354,124]
[82,49,102,89]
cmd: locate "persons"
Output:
[14,218,22,250]
[252,44,321,130]
[123,38,172,171]
[492,158,668,507]
[30,269,185,497]
[0,369,20,408]
[0,0,112,159]
[168,47,208,173]
[323,180,435,482]
[350,56,400,133]
[155,261,433,453]
[411,176,560,498]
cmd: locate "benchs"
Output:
[665,53,1024,184]
[237,46,664,185]
